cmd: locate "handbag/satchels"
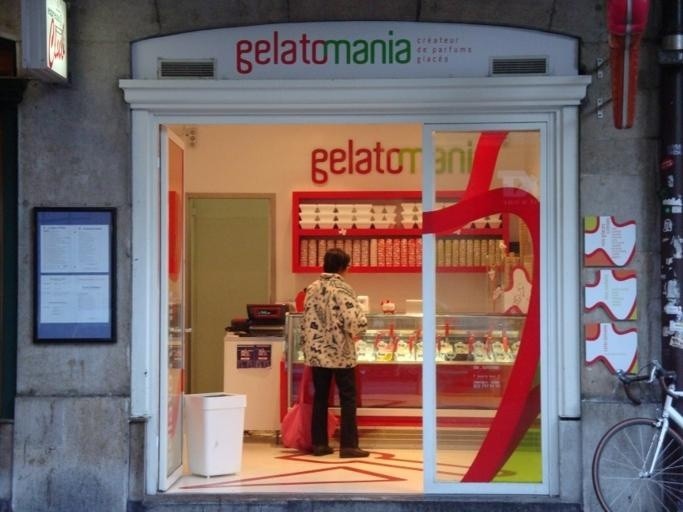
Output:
[281,365,339,450]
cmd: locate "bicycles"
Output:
[591,359,683,512]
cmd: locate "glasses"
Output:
[347,265,350,266]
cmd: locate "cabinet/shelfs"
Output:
[291,190,511,273]
[286,311,525,428]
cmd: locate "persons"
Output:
[299,247,371,459]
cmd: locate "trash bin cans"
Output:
[184,392,247,478]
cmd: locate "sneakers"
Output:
[313,443,333,456]
[340,448,370,458]
